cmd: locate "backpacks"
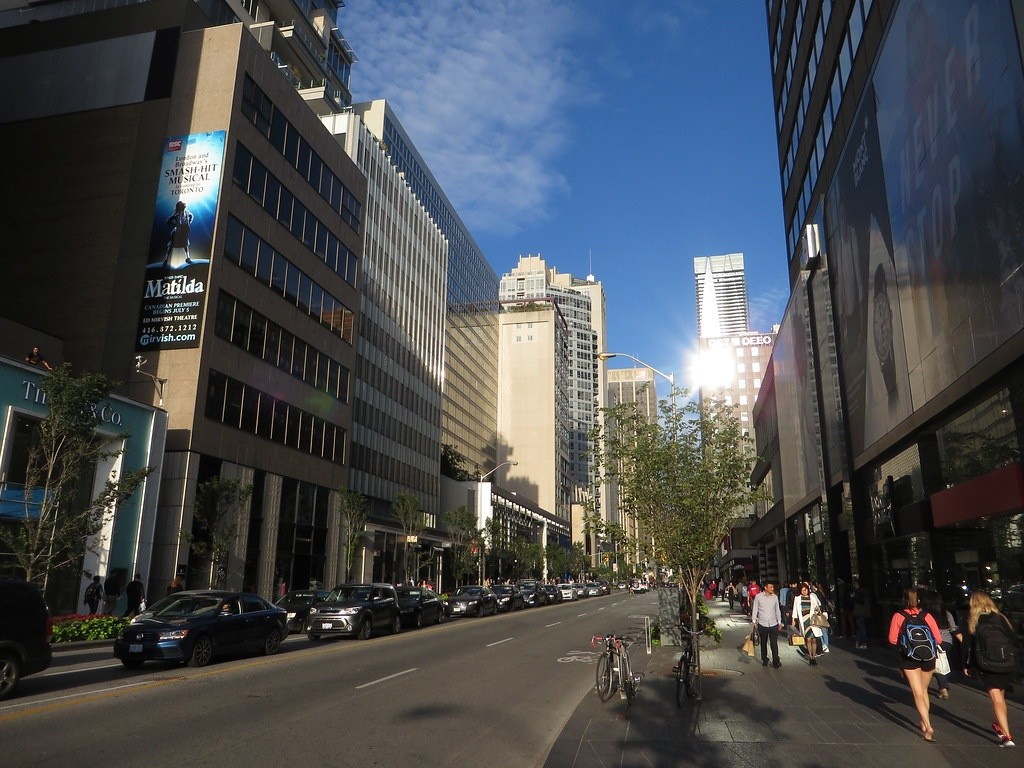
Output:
[84,582,97,603]
[895,610,938,663]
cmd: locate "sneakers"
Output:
[991,722,1006,739]
[999,736,1015,748]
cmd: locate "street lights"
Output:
[598,352,677,444]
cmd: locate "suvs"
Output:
[303,583,402,642]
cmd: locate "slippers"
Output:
[922,735,936,742]
[918,722,927,732]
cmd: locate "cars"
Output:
[395,586,449,629]
[114,588,291,667]
[594,580,611,596]
[442,585,498,617]
[0,579,54,700]
[572,583,590,599]
[617,581,628,590]
[556,583,578,601]
[275,590,331,634]
[630,578,679,594]
[513,579,550,608]
[587,582,605,598]
[545,584,564,605]
[491,585,525,613]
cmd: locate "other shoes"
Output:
[762,660,768,667]
[809,644,830,657]
[938,691,949,700]
[962,668,971,677]
[971,611,1018,673]
[812,659,818,666]
[773,662,782,668]
[809,659,813,666]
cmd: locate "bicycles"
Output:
[671,624,709,708]
[591,632,641,707]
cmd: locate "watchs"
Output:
[872,263,897,392]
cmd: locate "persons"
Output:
[276,577,286,596]
[25,345,52,371]
[961,589,1017,749]
[888,589,942,742]
[221,601,234,614]
[397,577,432,590]
[122,574,145,616]
[85,575,103,615]
[921,591,964,701]
[700,578,865,667]
[483,576,517,588]
[166,575,183,596]
[102,568,121,615]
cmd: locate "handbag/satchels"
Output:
[933,644,951,675]
[138,598,147,613]
[742,627,759,657]
[786,624,806,646]
[820,636,829,653]
[809,607,830,628]
